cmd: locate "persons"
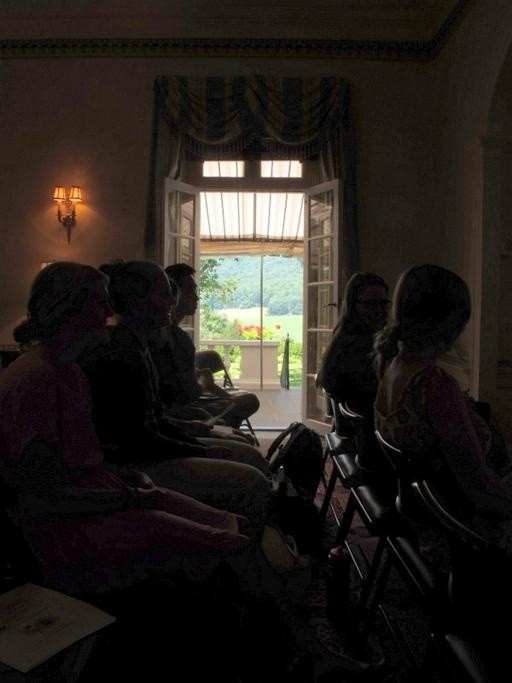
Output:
[3,261,274,594]
[312,271,394,483]
[371,264,511,536]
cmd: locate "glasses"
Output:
[356,297,391,314]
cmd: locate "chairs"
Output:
[195,350,260,447]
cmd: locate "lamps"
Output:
[51,185,83,241]
